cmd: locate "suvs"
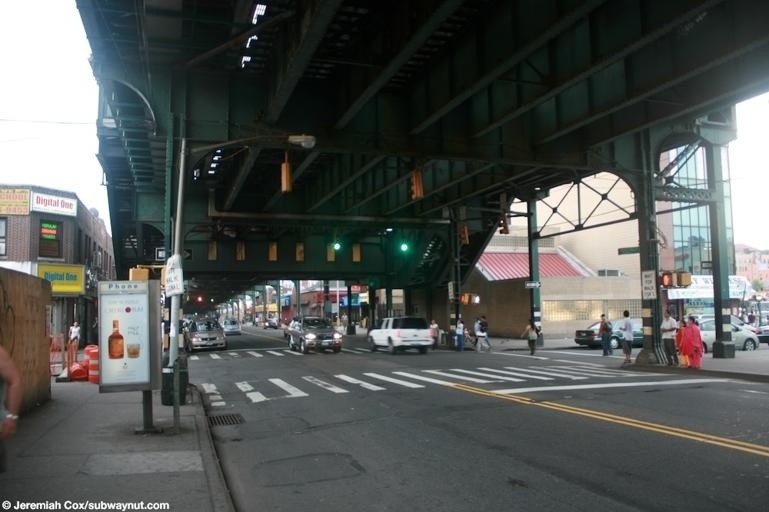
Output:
[367,316,435,354]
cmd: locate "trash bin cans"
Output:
[161,366,189,406]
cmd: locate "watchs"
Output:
[5,411,19,421]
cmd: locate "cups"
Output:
[127,344,140,358]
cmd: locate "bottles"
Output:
[108,319,125,360]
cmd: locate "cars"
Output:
[284,316,343,354]
[263,318,279,330]
[185,319,242,353]
[574,312,769,351]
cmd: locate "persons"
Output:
[676,320,690,369]
[67,320,82,353]
[520,318,540,357]
[659,308,679,367]
[747,310,756,326]
[341,312,348,331]
[451,315,491,353]
[742,311,750,324]
[618,310,634,364]
[1,346,25,480]
[597,314,615,358]
[429,318,438,350]
[91,316,98,344]
[685,316,704,369]
